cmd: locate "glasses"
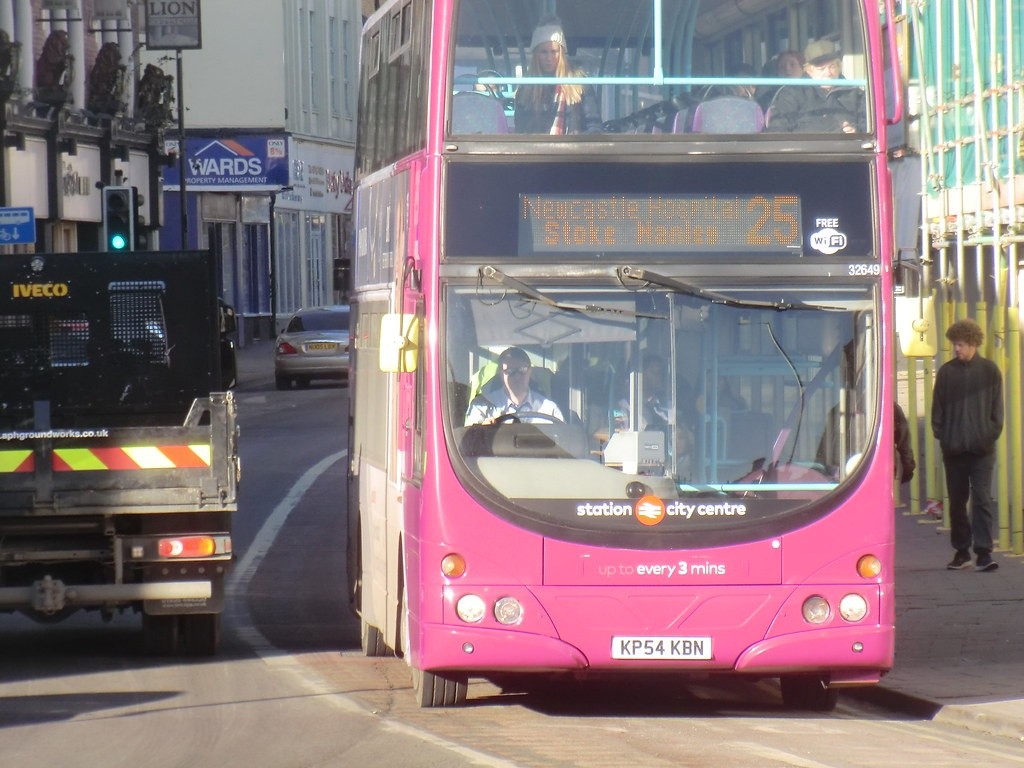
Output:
[500,365,529,375]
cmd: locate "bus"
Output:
[358,1,926,708]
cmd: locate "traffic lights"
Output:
[101,186,147,252]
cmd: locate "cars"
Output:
[274,303,351,389]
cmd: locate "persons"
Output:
[465,347,565,425]
[614,355,747,470]
[514,13,604,135]
[894,402,917,482]
[930,319,1004,571]
[725,40,867,133]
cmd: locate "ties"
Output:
[510,404,525,423]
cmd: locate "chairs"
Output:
[449,75,811,135]
[489,368,554,403]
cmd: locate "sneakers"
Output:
[975,556,999,571]
[947,551,972,569]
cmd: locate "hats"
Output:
[803,39,842,65]
[529,14,568,52]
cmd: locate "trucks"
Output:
[1,250,243,660]
